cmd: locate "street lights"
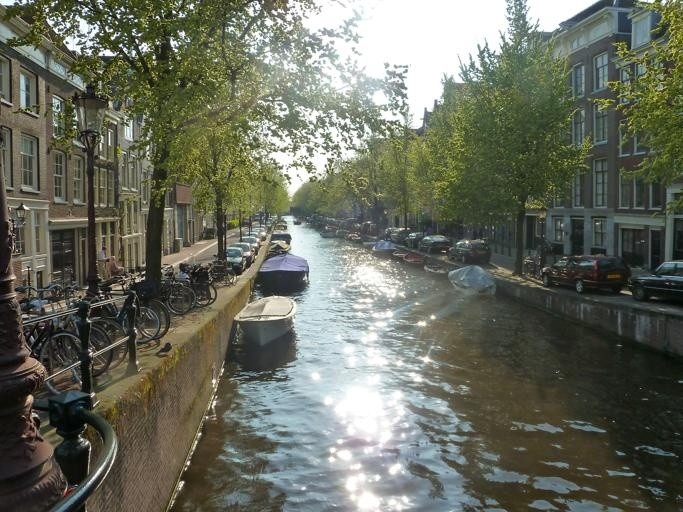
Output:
[538,205,547,265]
[70,81,110,297]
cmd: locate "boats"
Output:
[233,294,298,346]
[372,239,396,251]
[257,218,309,289]
[320,225,362,242]
[293,218,302,225]
[305,216,313,224]
[392,249,497,296]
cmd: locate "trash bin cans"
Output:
[206,228,216,239]
[174,238,183,253]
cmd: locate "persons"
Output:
[110,255,124,275]
[97,246,111,280]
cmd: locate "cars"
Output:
[448,238,492,265]
[541,255,683,301]
[313,213,376,233]
[214,216,276,275]
[384,225,450,254]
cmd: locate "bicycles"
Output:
[13,260,237,396]
